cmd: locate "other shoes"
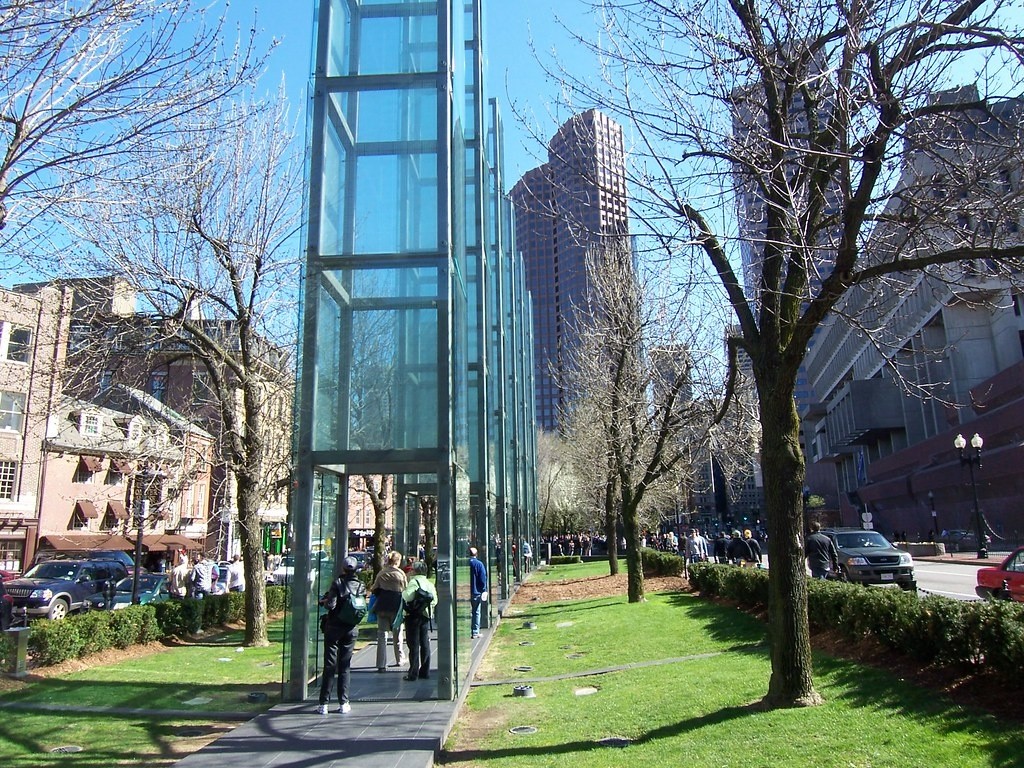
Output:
[472,633,484,639]
[403,676,416,681]
[418,675,429,679]
[377,668,386,673]
[397,663,408,668]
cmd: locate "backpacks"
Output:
[402,581,434,633]
[331,594,367,637]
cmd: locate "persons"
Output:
[469,547,488,639]
[166,552,245,600]
[640,528,762,568]
[497,538,533,586]
[568,540,575,556]
[402,556,416,574]
[927,527,948,536]
[371,551,409,672]
[315,555,368,715]
[893,530,907,542]
[583,537,591,556]
[267,550,287,571]
[621,536,626,549]
[803,521,839,579]
[401,561,438,683]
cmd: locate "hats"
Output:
[744,529,752,538]
[732,530,741,537]
[343,556,357,569]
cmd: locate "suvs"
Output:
[807,529,913,589]
[2,558,130,625]
[32,549,148,582]
[273,550,329,585]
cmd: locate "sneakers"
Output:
[317,705,328,714]
[339,703,351,714]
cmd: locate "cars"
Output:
[0,582,16,631]
[79,574,171,614]
[974,546,1024,604]
[0,569,21,583]
[211,560,232,596]
[348,548,375,573]
[935,529,991,552]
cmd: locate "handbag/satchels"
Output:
[836,571,847,583]
[756,563,764,569]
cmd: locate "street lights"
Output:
[928,490,940,536]
[802,485,810,535]
[953,432,988,558]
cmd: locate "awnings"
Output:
[109,458,132,474]
[78,455,103,472]
[155,463,174,479]
[45,535,203,552]
[76,500,98,520]
[107,500,131,520]
